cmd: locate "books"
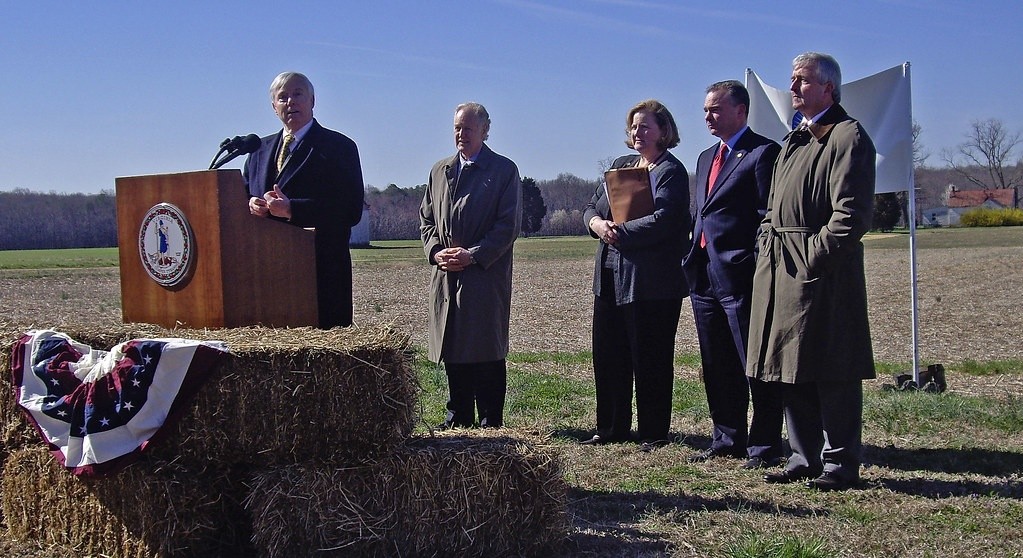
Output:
[602,167,656,226]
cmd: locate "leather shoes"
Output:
[579,433,628,446]
[690,446,743,463]
[762,469,815,483]
[640,442,662,452]
[744,456,781,470]
[805,471,859,490]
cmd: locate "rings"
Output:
[266,205,270,209]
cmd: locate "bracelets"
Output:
[285,199,292,222]
[591,216,602,226]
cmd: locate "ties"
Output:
[277,134,293,171]
[800,123,808,130]
[699,143,728,249]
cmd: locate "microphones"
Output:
[209,134,262,169]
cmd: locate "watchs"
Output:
[469,253,477,265]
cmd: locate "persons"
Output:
[242,71,365,326]
[418,102,522,431]
[576,100,692,454]
[680,80,786,469]
[744,50,877,492]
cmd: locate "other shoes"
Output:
[432,423,449,432]
